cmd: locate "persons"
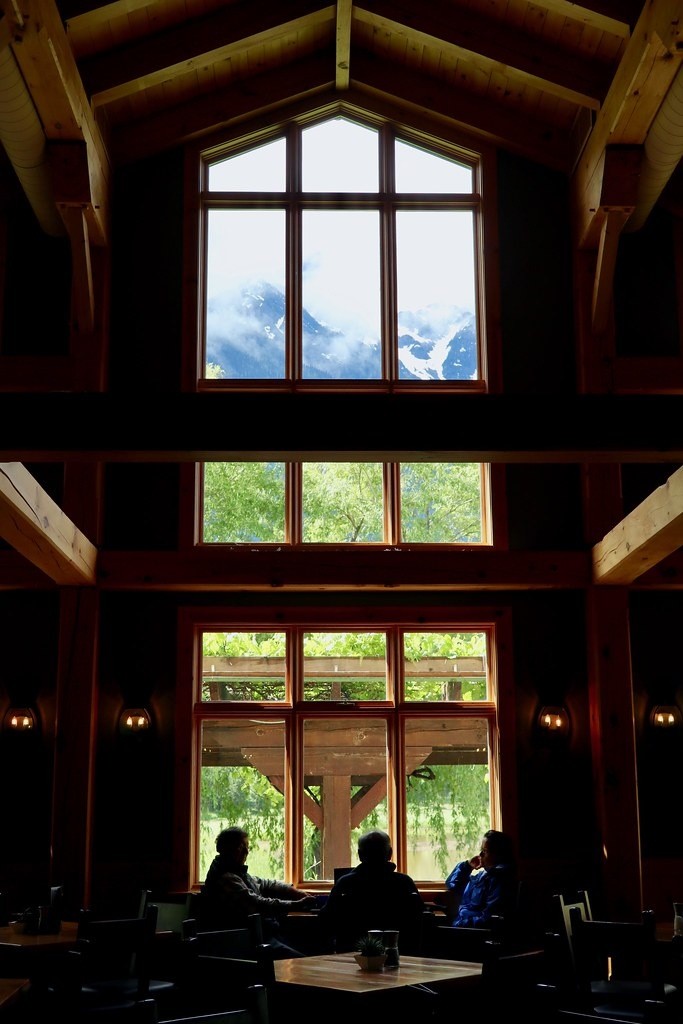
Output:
[443,827,520,931]
[315,831,438,957]
[199,828,317,1024]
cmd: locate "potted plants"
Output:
[8,912,25,934]
[352,936,388,972]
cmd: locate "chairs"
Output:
[0,884,679,1024]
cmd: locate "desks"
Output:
[0,978,32,1011]
[284,910,448,924]
[0,922,79,954]
[273,951,484,1001]
[651,923,674,950]
